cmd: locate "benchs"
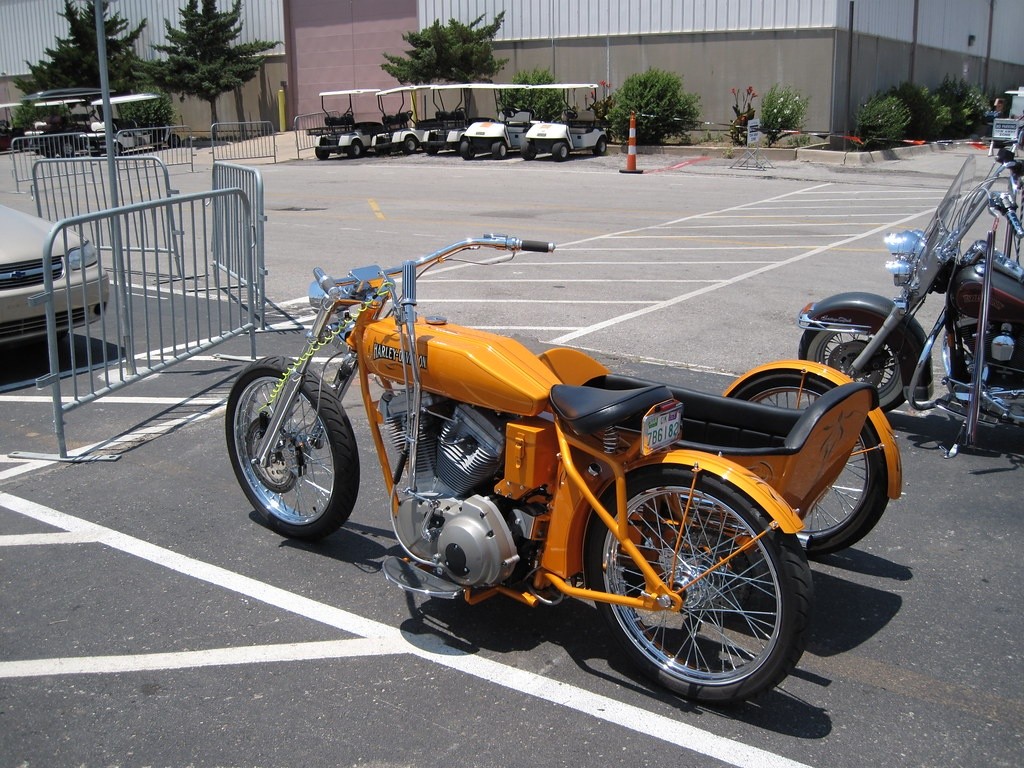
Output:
[465,117,495,125]
[498,110,532,132]
[417,119,441,130]
[569,111,596,134]
[351,122,382,133]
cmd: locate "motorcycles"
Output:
[794,121,1023,464]
[222,231,906,723]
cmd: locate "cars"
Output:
[0,202,110,358]
